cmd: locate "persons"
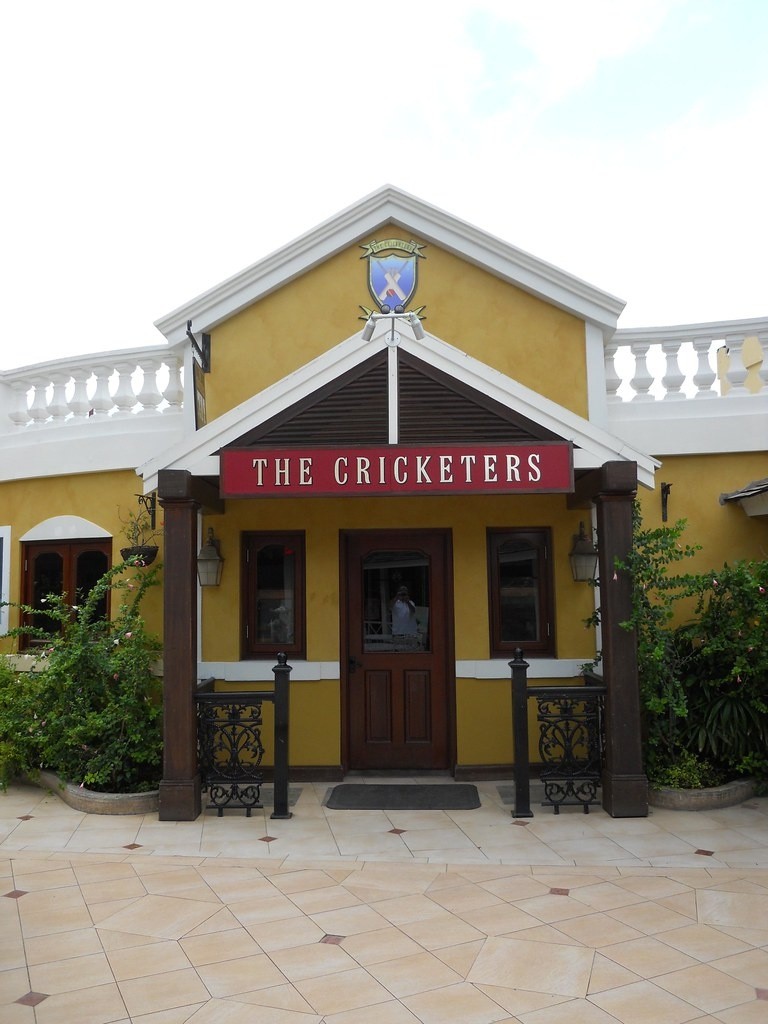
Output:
[386,585,417,637]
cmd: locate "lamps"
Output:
[568,520,598,583]
[360,303,426,348]
[197,526,226,588]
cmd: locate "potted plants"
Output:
[116,503,164,566]
